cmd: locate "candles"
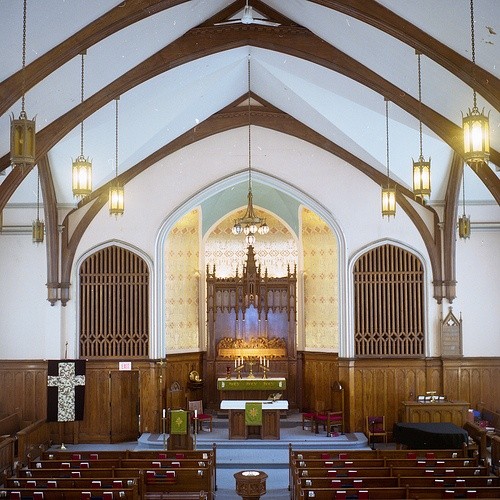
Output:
[260,356,262,364]
[195,410,197,417]
[238,358,240,366]
[235,360,236,368]
[163,409,165,417]
[263,357,265,366]
[267,360,269,367]
[242,357,243,364]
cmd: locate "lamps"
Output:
[411,50,431,204]
[457,170,471,244]
[108,97,125,221]
[31,170,44,247]
[70,48,93,204]
[232,55,269,244]
[459,0,491,175]
[8,0,39,175]
[381,96,396,220]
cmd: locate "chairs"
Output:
[365,416,387,446]
[314,381,344,438]
[302,400,326,433]
[166,379,193,433]
[188,400,213,433]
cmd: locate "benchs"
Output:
[0,413,500,500]
